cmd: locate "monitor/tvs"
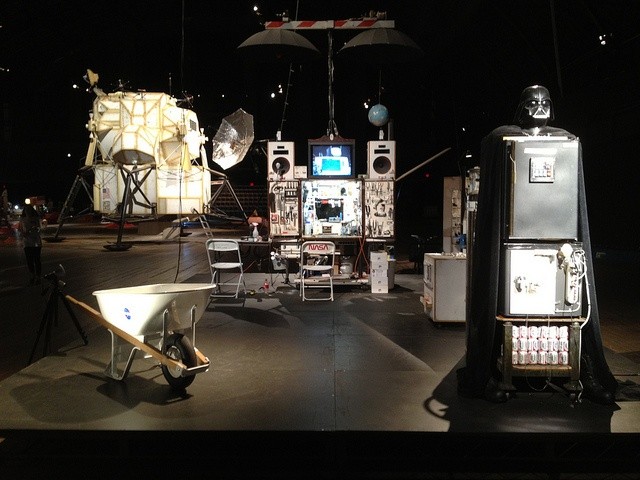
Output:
[307,138,355,179]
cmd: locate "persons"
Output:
[17,204,43,285]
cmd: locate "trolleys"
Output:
[64,280,217,391]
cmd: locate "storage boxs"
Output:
[370,261,388,271]
[370,278,389,285]
[370,270,388,277]
[372,286,388,294]
[370,253,387,261]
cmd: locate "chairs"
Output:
[297,240,335,302]
[206,238,247,299]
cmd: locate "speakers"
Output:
[367,141,396,180]
[267,140,294,181]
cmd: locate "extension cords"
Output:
[565,262,579,304]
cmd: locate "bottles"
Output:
[249,222,253,236]
[264,278,269,296]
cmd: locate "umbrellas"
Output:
[211,108,255,171]
[236,29,321,57]
[337,28,424,55]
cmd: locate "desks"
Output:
[209,235,272,292]
